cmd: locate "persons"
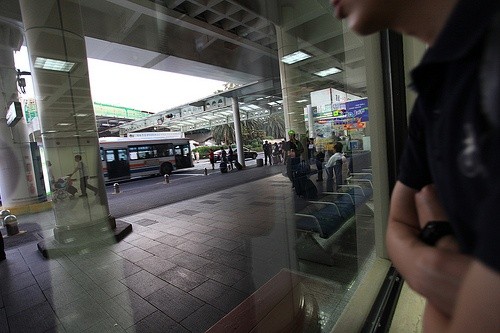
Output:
[209,149,214,170]
[325,142,346,172]
[69,155,98,197]
[219,147,228,164]
[227,146,234,171]
[48,161,54,182]
[330,0,500,333]
[315,133,325,182]
[263,140,287,165]
[284,131,304,187]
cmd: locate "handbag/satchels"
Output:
[302,176,319,201]
[282,164,288,177]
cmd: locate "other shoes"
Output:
[78,193,87,197]
[95,188,98,196]
[317,179,323,181]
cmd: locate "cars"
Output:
[209,147,258,163]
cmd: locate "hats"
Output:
[288,130,295,135]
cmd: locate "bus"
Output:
[99,136,193,184]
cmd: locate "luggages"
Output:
[235,160,243,170]
[294,159,306,195]
[220,162,227,173]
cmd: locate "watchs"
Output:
[420,219,454,247]
